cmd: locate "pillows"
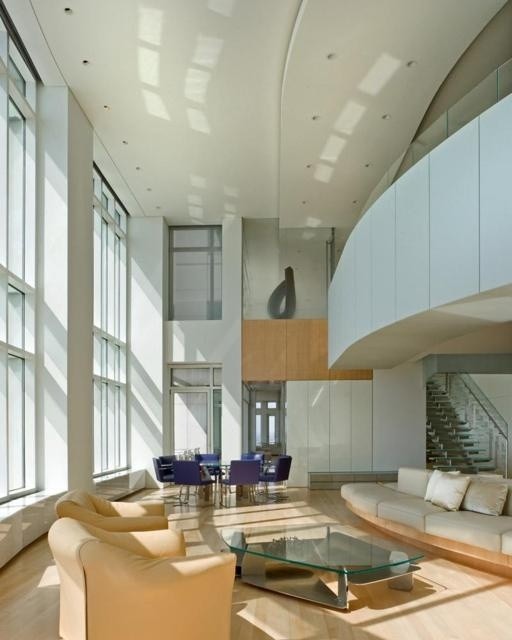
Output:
[460,482,509,517]
[424,469,460,501]
[431,473,471,511]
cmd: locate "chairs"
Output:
[48,517,237,640]
[55,488,168,531]
[152,453,292,509]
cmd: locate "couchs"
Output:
[341,468,512,570]
